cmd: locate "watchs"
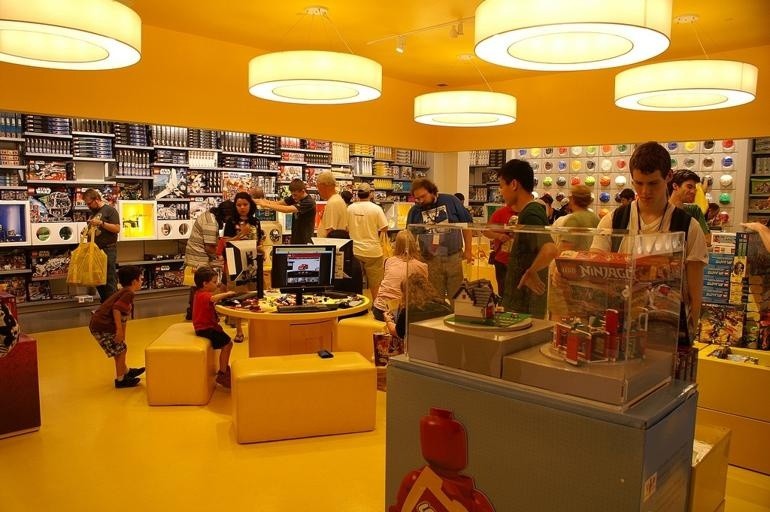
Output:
[99,221,106,228]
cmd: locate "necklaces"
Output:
[427,201,440,221]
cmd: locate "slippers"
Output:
[234,334,244,342]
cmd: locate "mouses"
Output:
[222,300,234,306]
[339,302,349,308]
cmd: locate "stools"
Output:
[231,352,378,445]
[144,323,221,406]
[337,311,387,362]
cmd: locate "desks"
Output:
[215,289,370,357]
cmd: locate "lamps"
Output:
[614,14,758,111]
[412,54,516,128]
[474,0,672,73]
[0,1,141,71]
[248,5,382,104]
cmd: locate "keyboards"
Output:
[223,291,257,301]
[277,303,336,313]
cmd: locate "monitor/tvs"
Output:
[308,237,354,282]
[272,245,336,304]
[226,239,267,300]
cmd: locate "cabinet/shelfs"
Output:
[457,149,507,220]
[385,221,699,511]
[697,344,770,477]
[0,110,435,333]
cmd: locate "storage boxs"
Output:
[688,422,731,512]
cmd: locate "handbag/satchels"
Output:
[488,252,495,264]
[373,332,404,366]
[66,241,108,287]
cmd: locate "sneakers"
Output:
[215,371,231,388]
[114,376,140,388]
[127,367,146,376]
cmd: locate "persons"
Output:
[536,172,721,250]
[183,199,239,318]
[224,192,265,343]
[317,172,389,300]
[88,265,147,388]
[742,223,770,253]
[482,200,520,300]
[407,177,476,303]
[191,266,248,389]
[375,271,454,341]
[456,193,471,216]
[498,160,560,320]
[251,178,316,244]
[590,142,710,330]
[82,188,121,301]
[373,229,428,321]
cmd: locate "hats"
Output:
[558,198,570,210]
[358,182,376,192]
